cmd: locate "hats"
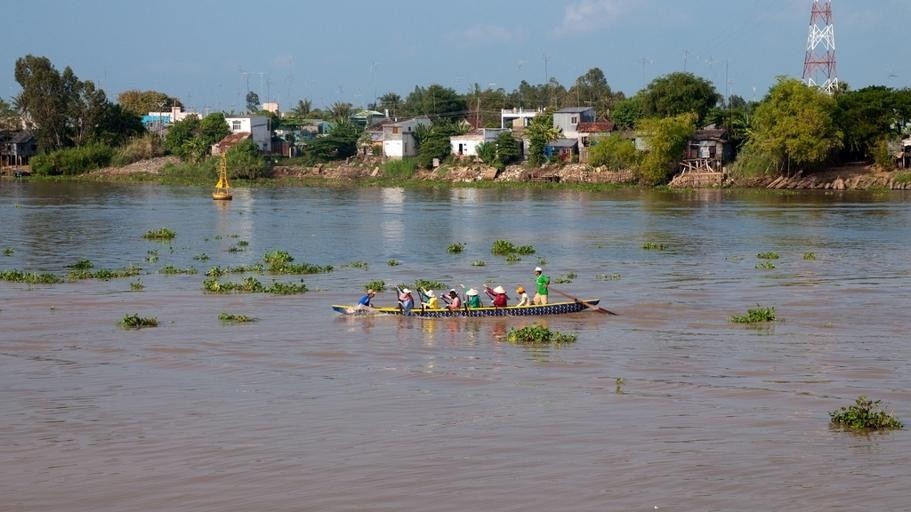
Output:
[517,287,525,293]
[534,268,542,272]
[426,291,433,297]
[400,294,409,300]
[449,289,456,297]
[466,289,478,295]
[493,286,505,294]
[403,288,411,293]
[367,289,375,294]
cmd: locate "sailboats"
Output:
[210,151,232,201]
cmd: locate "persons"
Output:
[396,286,529,321]
[359,288,375,307]
[534,267,551,304]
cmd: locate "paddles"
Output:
[548,285,615,315]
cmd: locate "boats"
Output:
[329,298,600,319]
